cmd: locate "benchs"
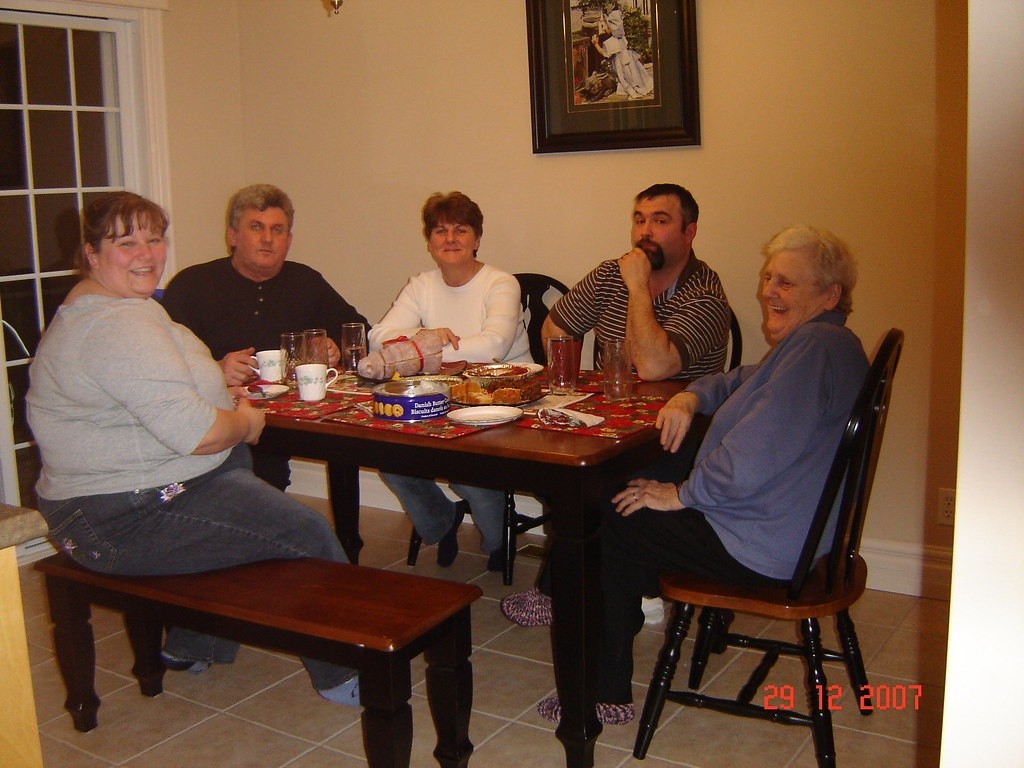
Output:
[32,548,489,768]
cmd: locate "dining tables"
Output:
[230,368,700,768]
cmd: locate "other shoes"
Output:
[536,695,635,726]
[486,546,505,573]
[436,513,466,566]
[161,649,211,672]
[318,674,359,707]
[500,586,554,627]
[641,596,665,625]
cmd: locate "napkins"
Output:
[534,365,646,393]
[322,396,498,439]
[517,392,673,440]
[458,363,489,379]
[232,388,374,421]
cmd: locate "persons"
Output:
[500,224,871,723]
[366,193,535,573]
[591,10,654,99]
[541,182,730,624]
[160,184,372,493]
[24,191,361,707]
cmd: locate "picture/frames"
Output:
[525,0,702,155]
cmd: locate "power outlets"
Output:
[935,487,956,526]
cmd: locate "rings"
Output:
[633,493,638,501]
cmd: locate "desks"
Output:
[0,499,52,768]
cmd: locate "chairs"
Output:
[634,326,905,768]
[406,272,583,588]
[592,307,745,374]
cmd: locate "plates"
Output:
[464,363,544,379]
[441,360,468,375]
[451,390,550,406]
[244,385,290,399]
[447,406,524,425]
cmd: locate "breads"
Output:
[449,379,543,405]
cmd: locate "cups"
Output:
[603,339,632,403]
[296,364,338,402]
[304,328,329,368]
[547,335,576,395]
[343,323,367,372]
[280,333,304,389]
[249,350,285,383]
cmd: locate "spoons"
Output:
[248,386,272,400]
